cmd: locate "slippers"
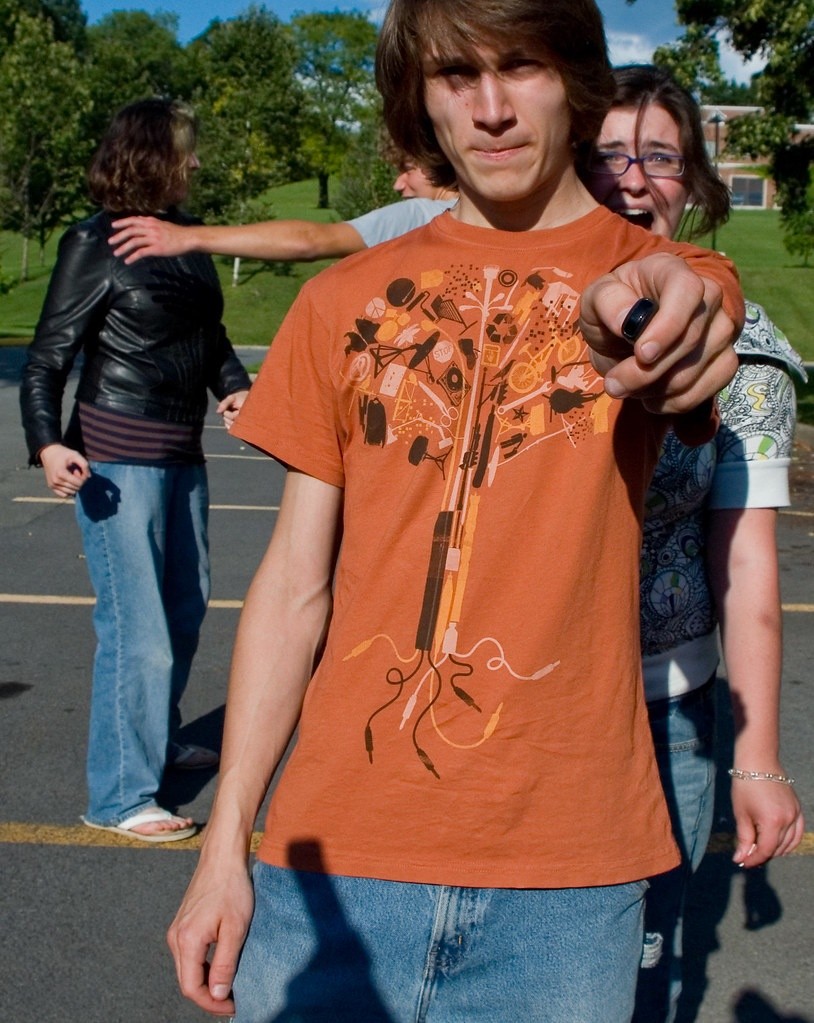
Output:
[83,809,197,843]
[166,741,219,767]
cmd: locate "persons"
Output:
[574,61,808,1023]
[109,117,460,266]
[20,96,255,843]
[164,1,747,1023]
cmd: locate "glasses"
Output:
[590,151,686,177]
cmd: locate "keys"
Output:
[622,298,660,341]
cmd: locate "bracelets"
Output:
[728,767,797,786]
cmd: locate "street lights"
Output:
[707,110,726,251]
